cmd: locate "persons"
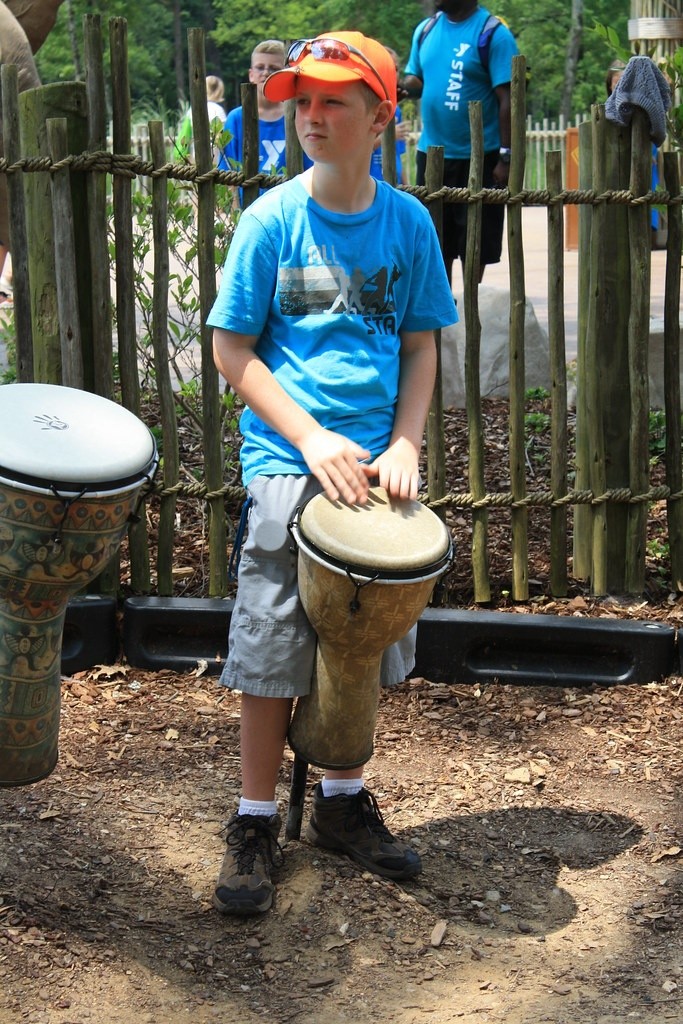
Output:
[212,31,460,913]
[172,0,528,316]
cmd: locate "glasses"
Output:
[250,65,278,74]
[282,39,390,104]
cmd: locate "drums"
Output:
[289,486,453,770]
[0,383,159,789]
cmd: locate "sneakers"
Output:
[213,813,284,914]
[309,784,423,883]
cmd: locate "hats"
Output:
[263,32,399,122]
